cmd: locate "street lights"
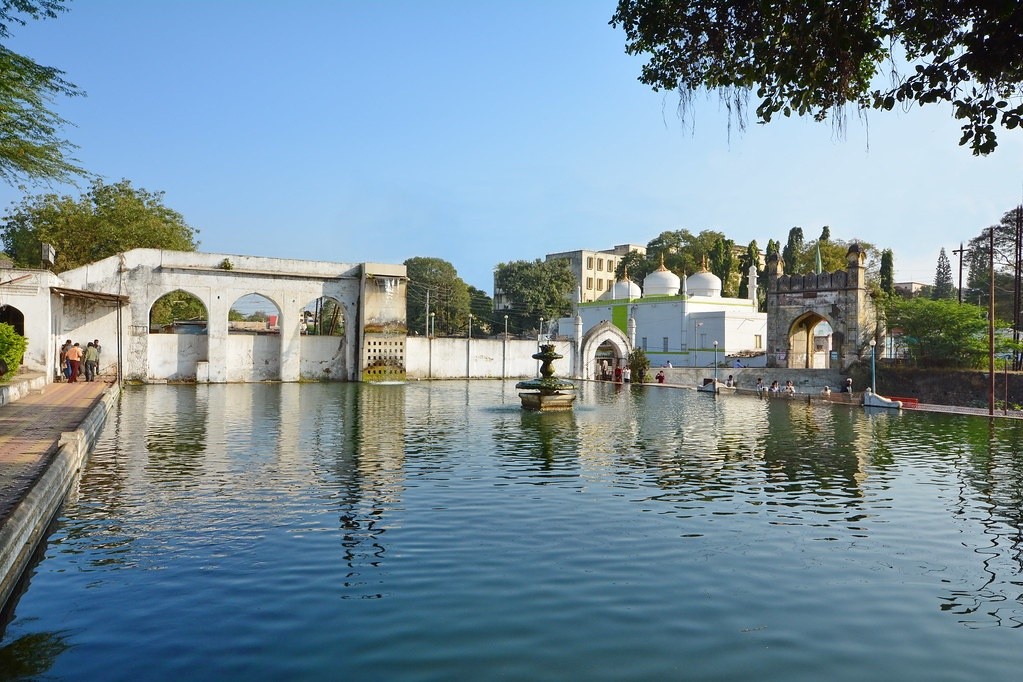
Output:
[430,312,435,336]
[869,339,876,393]
[713,341,718,378]
[503,315,509,338]
[539,317,544,334]
[468,313,473,338]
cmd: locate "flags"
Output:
[815,247,822,274]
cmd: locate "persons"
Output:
[666,360,673,369]
[656,371,665,384]
[59,340,102,383]
[734,359,745,368]
[601,361,631,383]
[725,374,853,396]
